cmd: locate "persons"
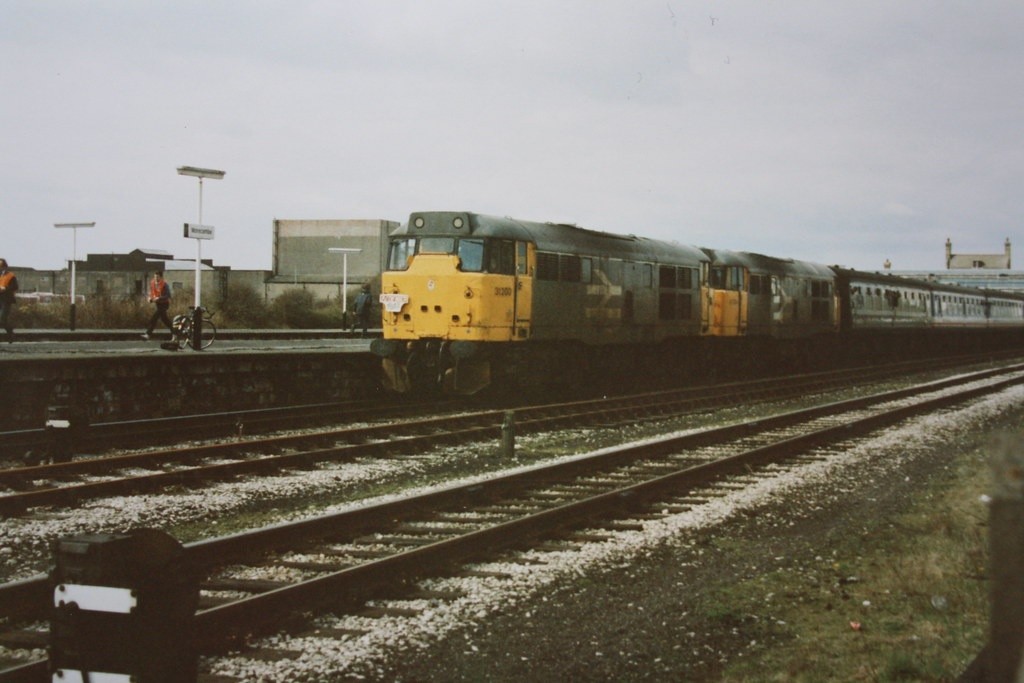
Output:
[351,283,373,338]
[139,271,173,341]
[0,257,19,343]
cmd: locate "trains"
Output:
[369,211,1024,400]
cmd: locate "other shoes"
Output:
[360,334,370,340]
[7,334,17,344]
[140,334,149,340]
[170,333,177,342]
[350,323,355,334]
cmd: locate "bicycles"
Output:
[177,305,216,350]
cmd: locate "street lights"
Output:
[54,222,95,332]
[177,166,225,350]
[328,248,364,331]
